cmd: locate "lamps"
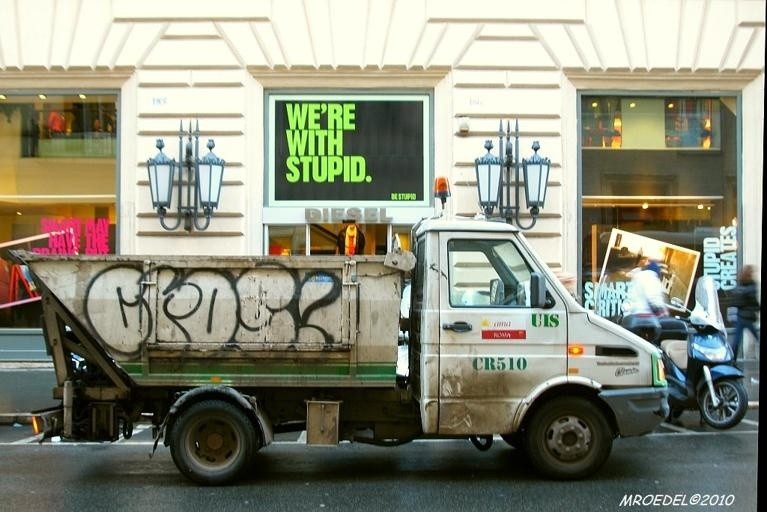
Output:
[472,119,552,231]
[143,118,225,233]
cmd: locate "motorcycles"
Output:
[623,274,748,429]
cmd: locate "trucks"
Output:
[3,212,670,487]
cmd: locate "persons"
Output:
[621,262,670,319]
[728,265,760,365]
